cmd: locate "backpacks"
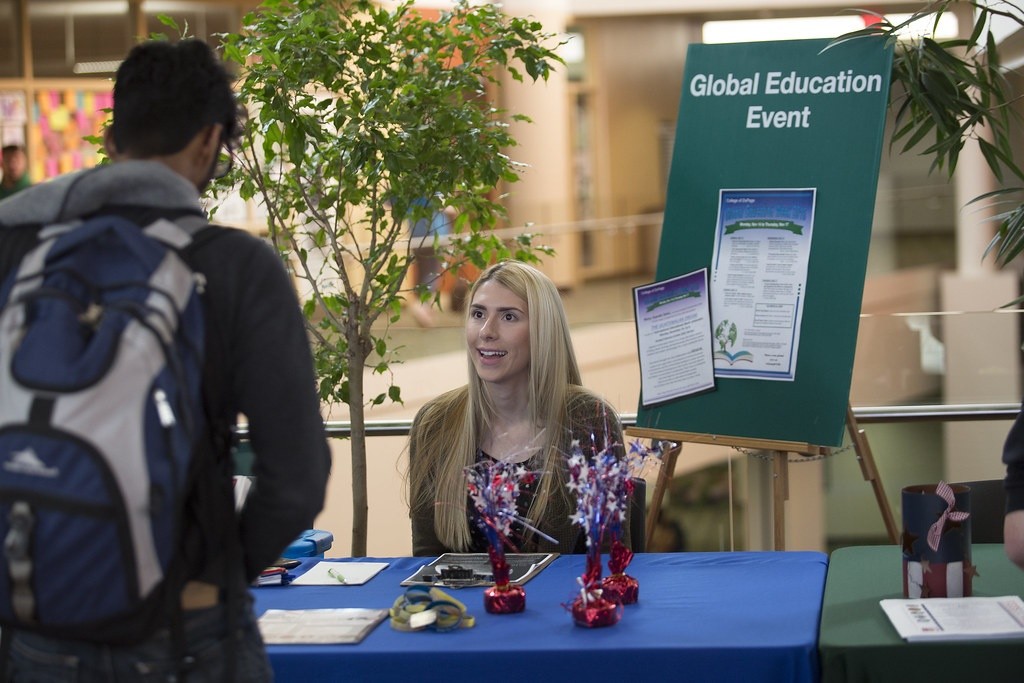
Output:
[0,215,216,635]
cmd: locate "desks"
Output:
[238,550,831,683]
[819,539,1024,682]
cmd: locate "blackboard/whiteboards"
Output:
[635,36,897,449]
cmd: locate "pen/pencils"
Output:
[328,568,347,585]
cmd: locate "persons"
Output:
[1000,398,1024,569]
[407,256,631,559]
[0,138,31,196]
[0,37,333,683]
